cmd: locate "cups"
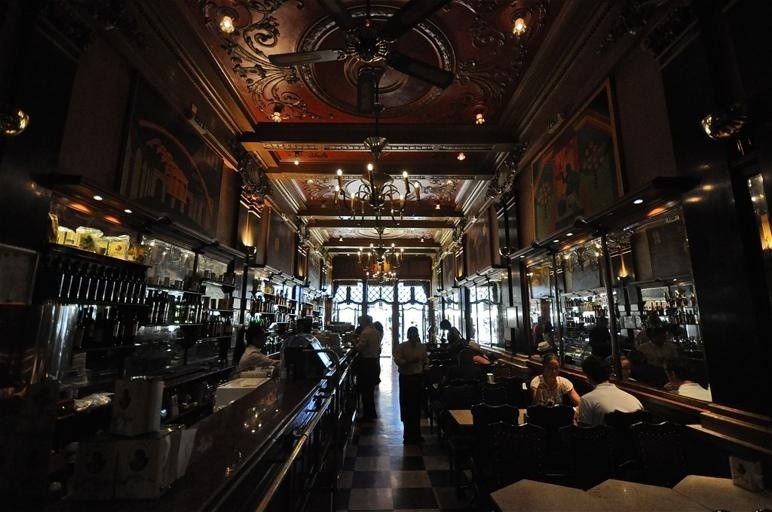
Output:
[149,277,160,286]
[175,280,183,289]
[486,372,494,383]
[95,246,105,255]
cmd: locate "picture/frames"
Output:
[466,208,493,276]
[115,70,224,237]
[531,74,628,242]
[265,207,296,275]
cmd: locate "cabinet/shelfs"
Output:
[565,305,699,351]
[37,242,322,425]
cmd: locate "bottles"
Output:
[649,292,697,324]
[250,315,281,355]
[201,312,233,338]
[85,305,139,344]
[205,270,222,283]
[142,287,203,323]
[202,297,228,310]
[51,256,147,305]
[564,296,607,325]
[255,285,295,314]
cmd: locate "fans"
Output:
[269,1,455,113]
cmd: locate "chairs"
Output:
[424,343,700,491]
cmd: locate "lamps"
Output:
[499,247,511,257]
[0,109,30,137]
[701,103,752,156]
[358,222,403,286]
[329,134,426,236]
[513,18,527,36]
[219,16,235,34]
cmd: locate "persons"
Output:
[530,353,580,416]
[350,316,384,424]
[444,342,490,385]
[529,316,679,387]
[571,355,645,444]
[394,325,428,444]
[230,325,282,379]
[440,319,460,346]
